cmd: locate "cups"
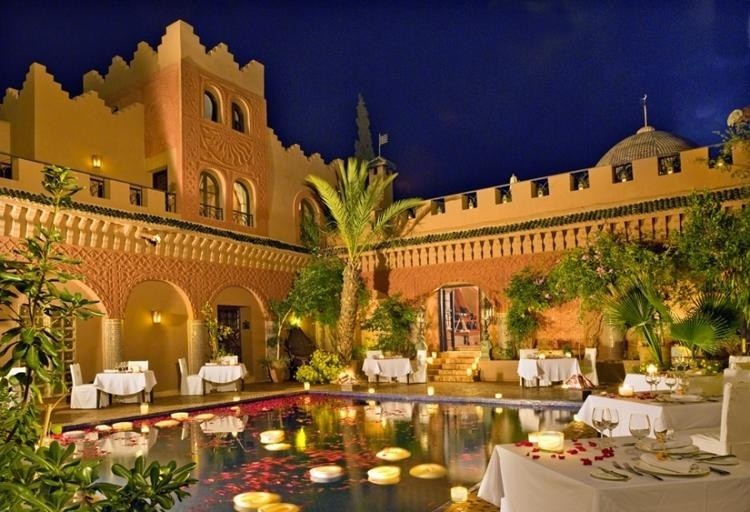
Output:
[628,412,651,439]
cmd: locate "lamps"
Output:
[92,154,102,167]
[152,309,162,325]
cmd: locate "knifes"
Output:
[598,460,664,482]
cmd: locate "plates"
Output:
[591,468,632,481]
[635,437,739,479]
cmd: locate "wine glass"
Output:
[591,406,620,446]
[653,417,675,452]
[645,369,691,399]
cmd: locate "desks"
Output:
[95,370,157,408]
[199,363,248,395]
[478,348,750,512]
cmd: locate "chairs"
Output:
[360,348,427,386]
[71,362,107,410]
[179,356,209,396]
[116,361,149,404]
